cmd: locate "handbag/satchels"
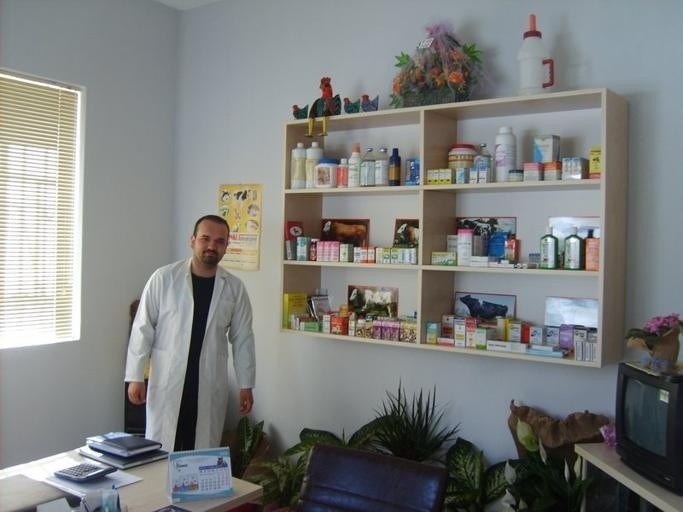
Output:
[402,82,468,108]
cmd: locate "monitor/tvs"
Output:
[613,364,683,497]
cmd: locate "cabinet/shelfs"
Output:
[279,90,630,369]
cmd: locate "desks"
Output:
[573,443,683,512]
[0,449,265,512]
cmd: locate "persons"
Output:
[122,214,258,451]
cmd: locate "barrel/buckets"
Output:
[518,31,556,95]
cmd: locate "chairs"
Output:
[295,444,450,512]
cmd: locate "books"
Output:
[86,431,162,458]
[78,445,169,472]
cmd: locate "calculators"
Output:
[54,462,116,482]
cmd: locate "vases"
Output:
[403,89,468,107]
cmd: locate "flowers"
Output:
[390,21,485,107]
[625,312,683,373]
